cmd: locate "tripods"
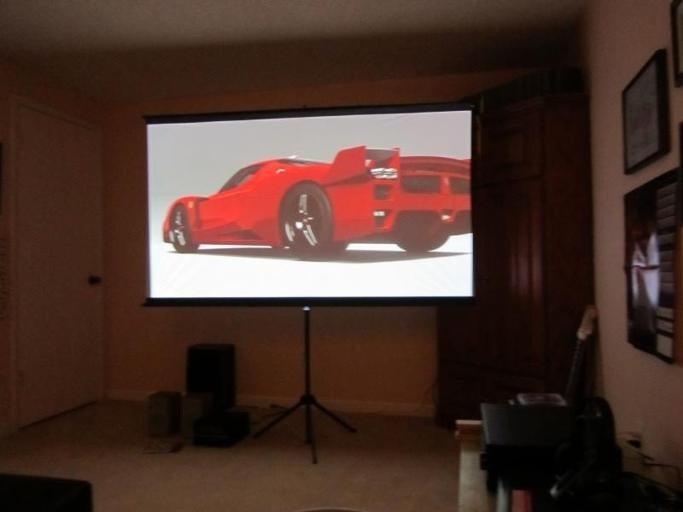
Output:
[252,307,356,463]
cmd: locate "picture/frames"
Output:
[621,1,683,366]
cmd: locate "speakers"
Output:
[186,342,235,409]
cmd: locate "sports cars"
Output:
[162,144,472,262]
